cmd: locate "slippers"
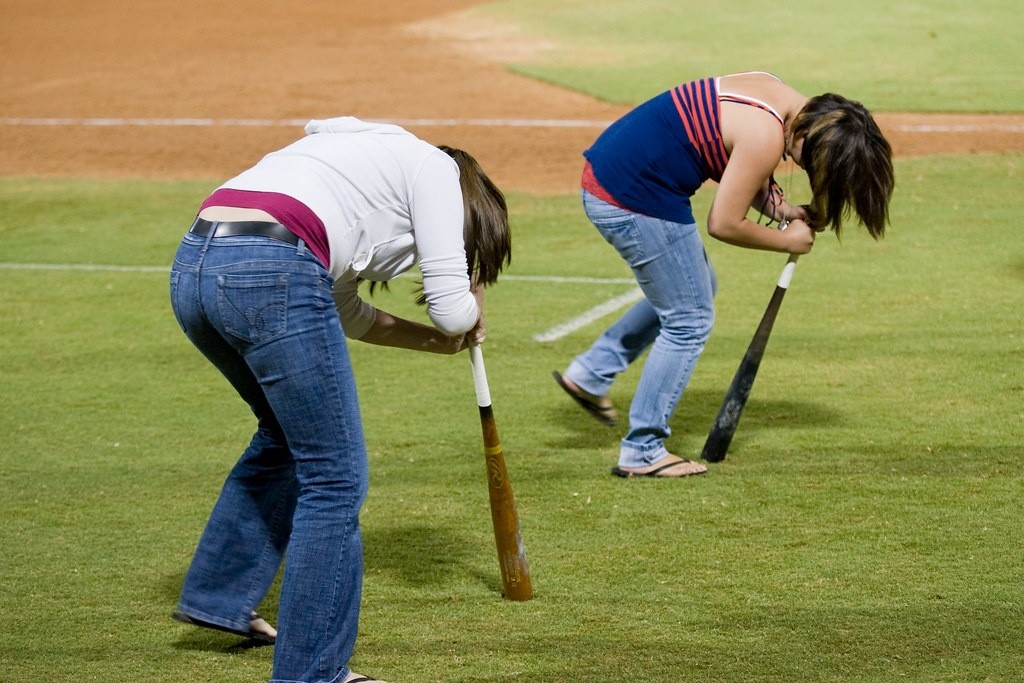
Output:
[552,370,615,427]
[171,612,276,644]
[346,675,375,683]
[611,457,707,478]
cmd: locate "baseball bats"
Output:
[468,334,534,601]
[699,251,801,461]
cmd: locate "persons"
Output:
[551,69,895,478]
[170,117,512,683]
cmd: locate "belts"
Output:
[189,216,308,247]
